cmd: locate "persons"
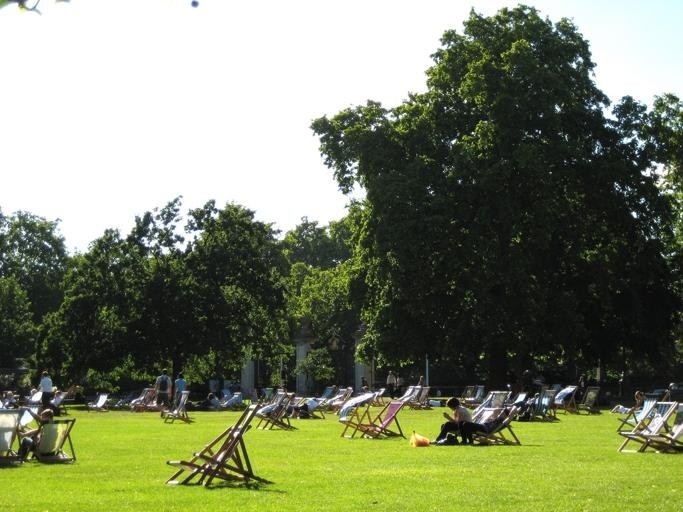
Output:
[37,370,54,408]
[360,376,368,386]
[287,398,319,419]
[8,406,57,461]
[386,370,396,399]
[517,392,539,422]
[154,368,171,406]
[208,392,221,409]
[3,390,19,409]
[174,371,185,402]
[290,394,300,405]
[635,390,646,407]
[429,397,473,444]
[49,390,61,407]
[131,389,154,405]
[418,375,427,388]
[458,405,518,444]
[359,386,368,393]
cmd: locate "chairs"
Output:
[165,402,272,489]
[87,388,193,423]
[616,384,683,453]
[256,386,434,439]
[441,384,604,446]
[0,391,75,465]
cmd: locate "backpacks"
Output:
[159,377,169,391]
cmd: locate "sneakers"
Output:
[430,440,437,445]
[460,439,474,445]
